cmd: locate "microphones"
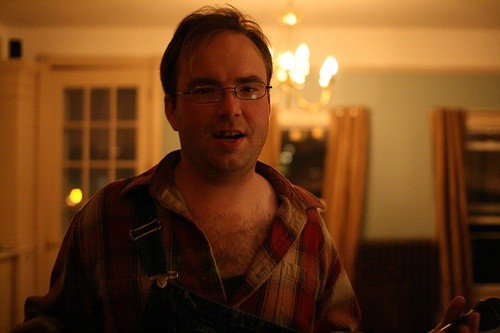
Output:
[437,298,500,333]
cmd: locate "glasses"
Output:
[172,79,273,103]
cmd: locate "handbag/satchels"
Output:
[139,279,296,333]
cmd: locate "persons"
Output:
[12,4,480,333]
